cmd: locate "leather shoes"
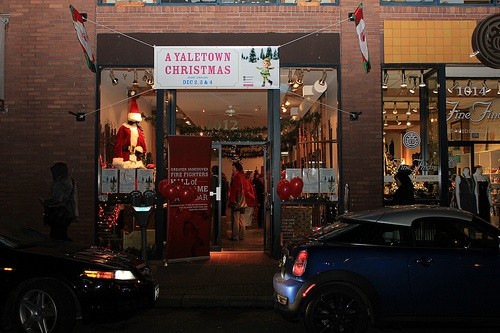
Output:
[228,237,244,241]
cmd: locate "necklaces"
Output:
[463,173,474,194]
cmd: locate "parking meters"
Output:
[134,203,152,263]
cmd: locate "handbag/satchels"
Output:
[42,199,75,227]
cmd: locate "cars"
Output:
[0,237,161,333]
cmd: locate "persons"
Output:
[456,166,477,215]
[383,171,416,206]
[472,165,492,223]
[42,162,75,240]
[211,163,264,244]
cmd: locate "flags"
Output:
[353,5,372,75]
[70,6,97,73]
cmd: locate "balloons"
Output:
[158,179,195,206]
[277,177,304,201]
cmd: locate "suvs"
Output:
[270,206,500,333]
